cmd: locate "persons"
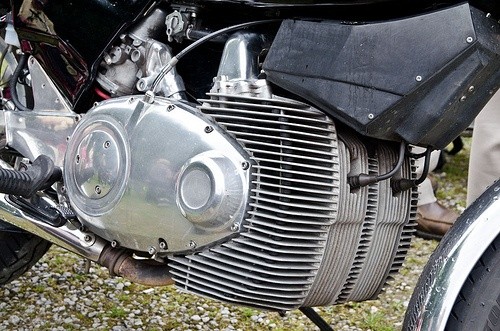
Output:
[411,145,463,242]
[47,136,179,204]
[465,90,499,212]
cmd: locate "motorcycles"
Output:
[0,0,500,331]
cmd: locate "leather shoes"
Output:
[412,201,457,242]
